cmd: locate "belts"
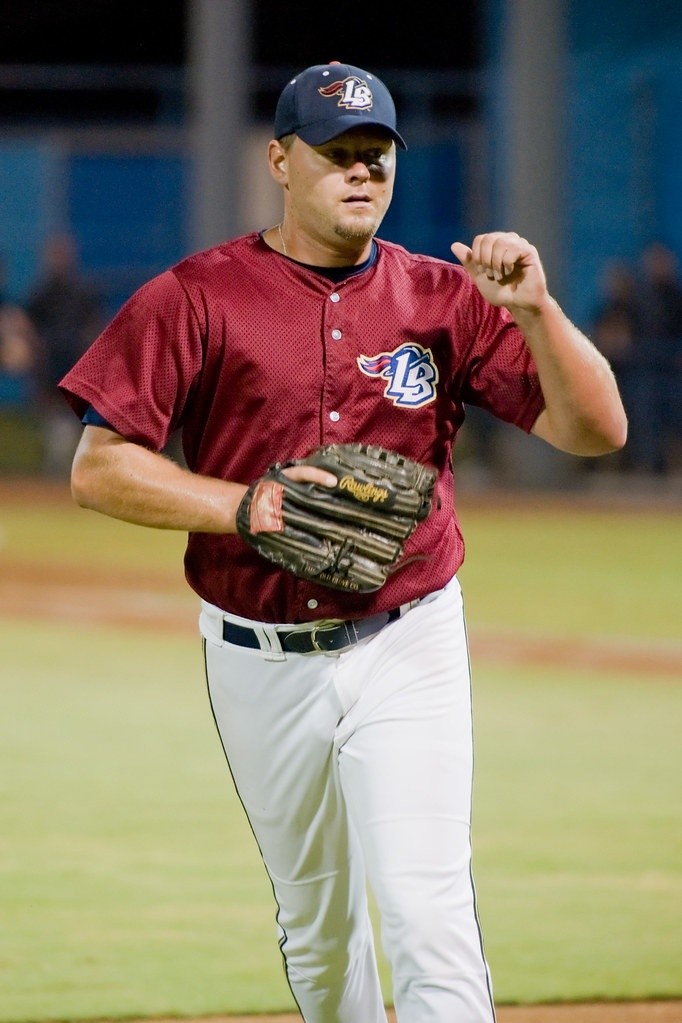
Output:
[223,597,426,654]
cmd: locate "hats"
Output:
[274,60,409,151]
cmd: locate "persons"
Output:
[62,61,627,1023]
[0,234,112,479]
[579,242,682,476]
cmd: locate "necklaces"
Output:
[279,223,287,254]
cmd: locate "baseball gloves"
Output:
[233,441,438,595]
